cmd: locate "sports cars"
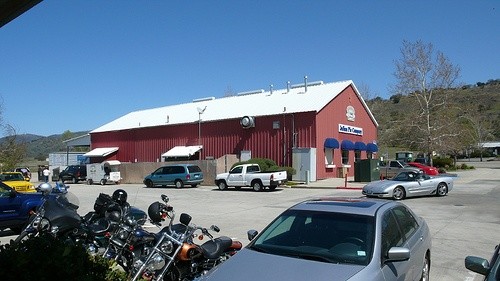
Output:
[362,172,453,201]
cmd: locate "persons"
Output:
[43,167,51,183]
[38,165,42,181]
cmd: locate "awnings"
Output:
[367,143,378,152]
[341,140,357,150]
[326,138,339,149]
[355,142,369,150]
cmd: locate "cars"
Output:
[406,162,438,176]
[193,197,432,281]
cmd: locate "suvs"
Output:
[59,165,87,184]
[0,172,57,233]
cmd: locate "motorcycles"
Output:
[15,183,243,281]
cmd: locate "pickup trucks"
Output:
[380,160,424,178]
[215,163,287,192]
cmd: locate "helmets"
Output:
[148,202,166,222]
[38,183,52,192]
[112,189,127,203]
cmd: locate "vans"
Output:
[143,164,204,189]
